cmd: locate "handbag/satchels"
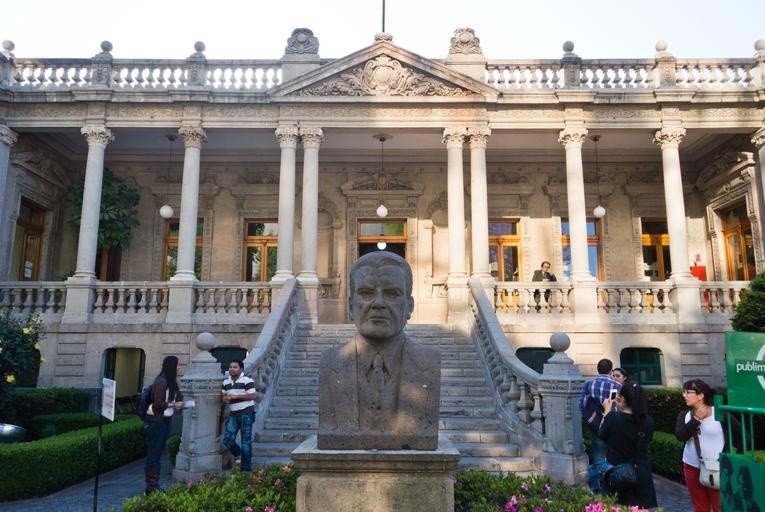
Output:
[584,399,603,432]
[699,459,721,491]
[607,464,638,495]
[138,386,152,420]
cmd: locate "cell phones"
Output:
[612,393,616,400]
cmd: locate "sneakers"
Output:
[234,455,241,466]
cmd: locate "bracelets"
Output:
[171,402,175,407]
[695,414,703,421]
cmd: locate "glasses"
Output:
[683,389,696,393]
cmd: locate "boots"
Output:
[144,466,165,496]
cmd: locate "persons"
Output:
[719,456,737,512]
[612,368,628,387]
[532,261,558,312]
[733,465,762,512]
[578,357,622,462]
[141,354,186,496]
[316,251,444,449]
[220,360,259,472]
[673,378,759,512]
[596,380,658,509]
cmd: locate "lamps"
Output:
[159,134,176,219]
[589,134,605,218]
[372,133,393,218]
[377,223,386,250]
